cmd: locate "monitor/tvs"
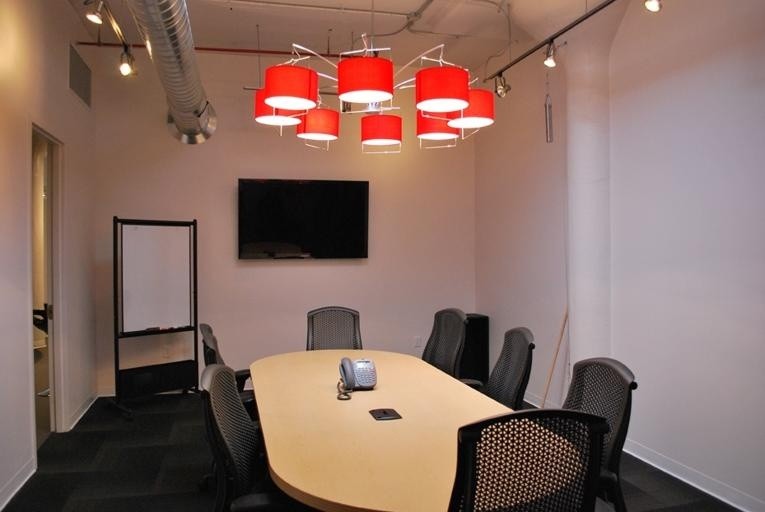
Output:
[237,178,370,261]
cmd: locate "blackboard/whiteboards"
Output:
[122,224,190,333]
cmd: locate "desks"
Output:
[249,350,582,511]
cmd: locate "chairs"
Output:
[199,323,255,401]
[559,357,635,512]
[201,363,282,512]
[448,408,605,512]
[306,306,362,351]
[420,307,468,379]
[478,326,535,409]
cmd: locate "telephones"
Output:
[336,356,378,400]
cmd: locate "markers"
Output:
[146,326,160,331]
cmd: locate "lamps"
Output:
[645,0,662,14]
[360,114,403,145]
[415,110,460,141]
[445,87,496,130]
[543,42,558,69]
[336,57,393,104]
[85,1,105,26]
[263,65,319,111]
[415,66,469,113]
[496,78,511,97]
[254,88,304,126]
[296,108,340,141]
[116,54,132,77]
